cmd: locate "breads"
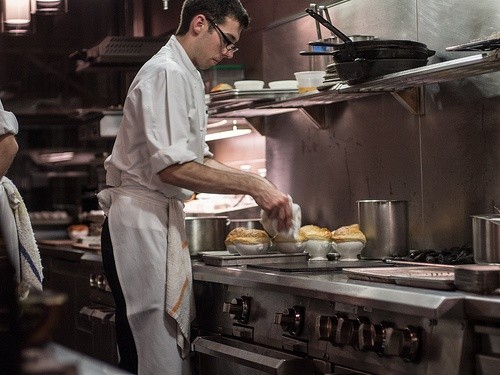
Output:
[210,83,232,91]
[67,224,89,231]
[225,224,367,245]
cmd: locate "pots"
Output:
[299,6,437,91]
[383,259,500,295]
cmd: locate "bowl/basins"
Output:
[211,83,233,92]
[234,80,264,89]
[68,225,88,239]
[226,240,365,261]
[268,79,297,90]
[293,70,325,93]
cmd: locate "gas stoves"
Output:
[187,259,499,375]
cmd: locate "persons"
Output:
[97,1,294,375]
[0,101,20,187]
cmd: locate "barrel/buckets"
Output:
[185,215,228,256]
[355,199,409,260]
[228,220,265,234]
[469,213,500,264]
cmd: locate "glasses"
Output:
[206,17,238,54]
